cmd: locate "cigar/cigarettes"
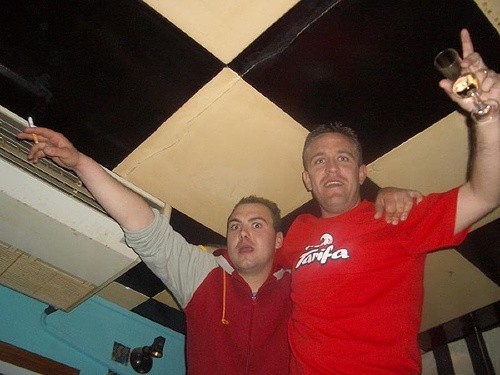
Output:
[29,117,39,144]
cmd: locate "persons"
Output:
[191,28,500,375]
[16,127,422,375]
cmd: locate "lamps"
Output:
[129,335,166,375]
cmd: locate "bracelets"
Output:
[198,245,207,253]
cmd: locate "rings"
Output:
[479,69,488,76]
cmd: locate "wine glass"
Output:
[434,49,500,125]
